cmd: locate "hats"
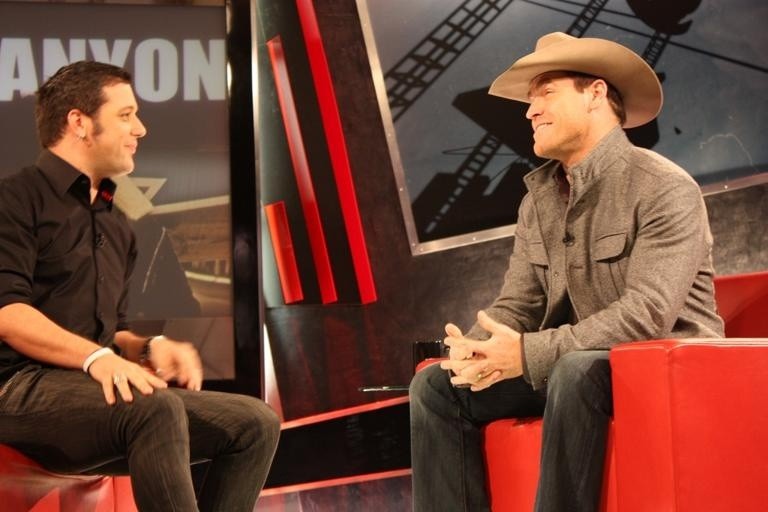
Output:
[488,31,664,129]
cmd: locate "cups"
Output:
[412,340,450,377]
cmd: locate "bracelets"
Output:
[144,333,166,360]
[83,346,114,373]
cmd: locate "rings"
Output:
[113,376,128,385]
[478,373,483,380]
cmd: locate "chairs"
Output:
[416,271,768,512]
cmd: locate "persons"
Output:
[409,30,725,512]
[0,60,280,511]
